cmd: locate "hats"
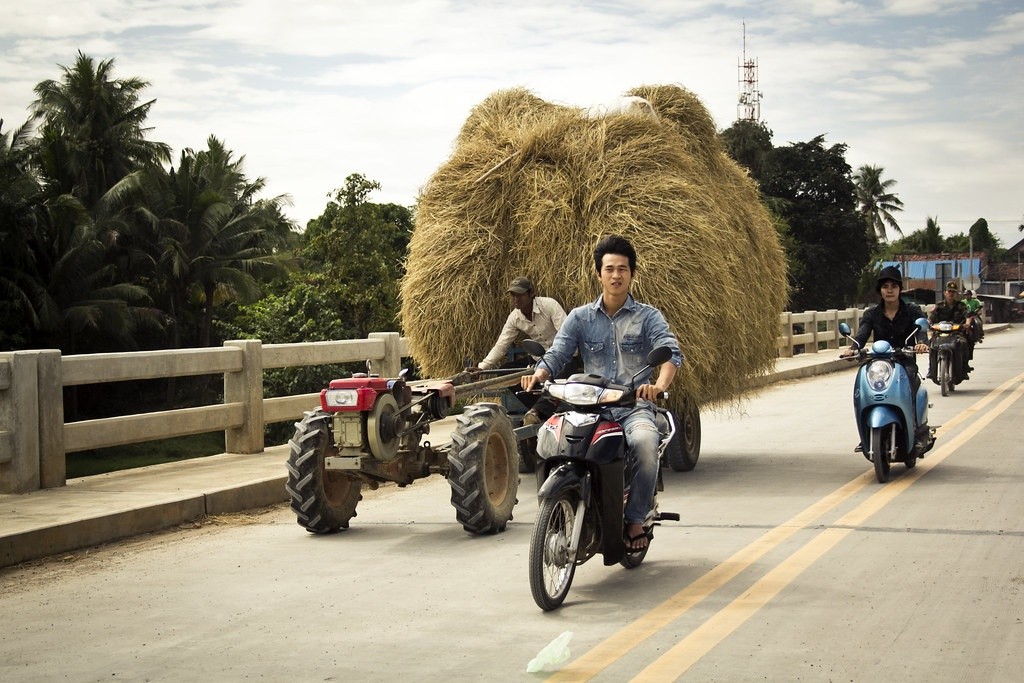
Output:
[945,281,958,292]
[878,266,903,283]
[963,289,972,294]
[505,277,535,295]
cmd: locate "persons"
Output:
[970,290,977,299]
[926,281,972,380]
[521,234,681,554]
[841,266,928,452]
[961,290,983,344]
[464,277,584,427]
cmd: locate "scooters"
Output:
[839,318,940,485]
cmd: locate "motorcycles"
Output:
[924,306,984,397]
[515,340,680,612]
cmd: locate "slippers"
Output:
[625,528,648,552]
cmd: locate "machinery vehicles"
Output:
[286,359,700,536]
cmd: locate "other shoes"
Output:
[914,425,929,448]
[925,371,931,378]
[977,338,982,343]
[962,373,969,380]
[854,442,863,452]
[522,411,541,427]
[967,365,974,372]
